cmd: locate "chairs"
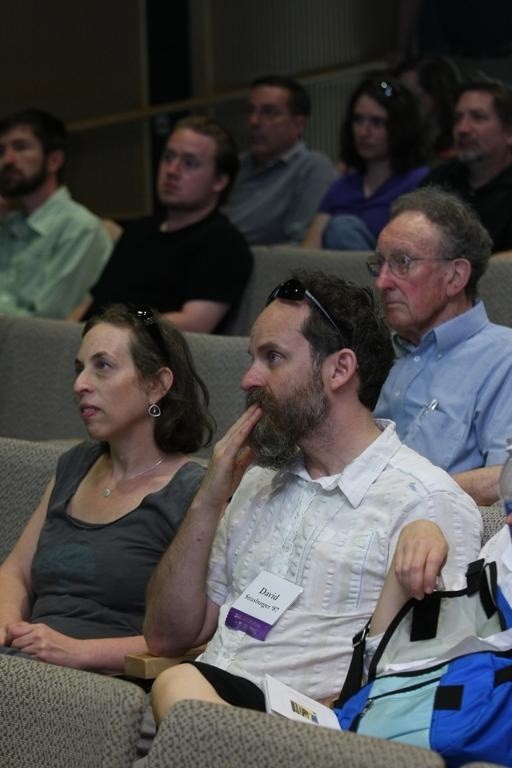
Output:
[2,652,149,767]
[211,242,511,344]
[0,312,265,455]
[3,437,79,560]
[136,698,445,768]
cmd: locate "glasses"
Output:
[123,303,178,379]
[363,250,457,279]
[265,278,349,354]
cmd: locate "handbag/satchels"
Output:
[328,561,512,767]
